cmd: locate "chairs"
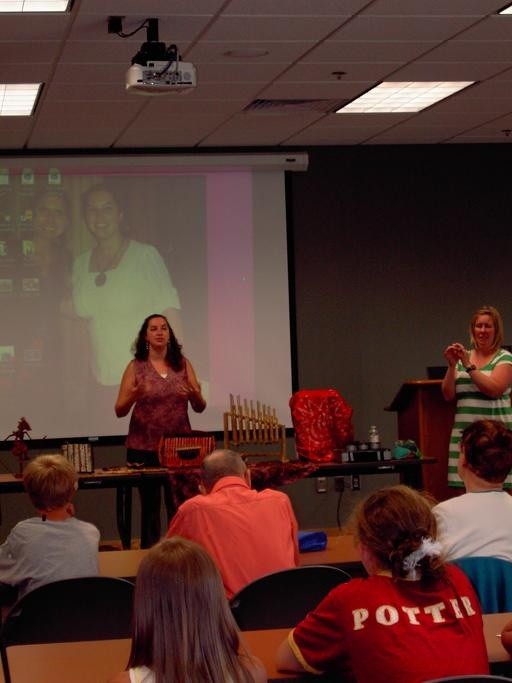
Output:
[452,556,512,614]
[1,577,138,643]
[228,565,351,630]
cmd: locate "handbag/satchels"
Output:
[298,530,327,551]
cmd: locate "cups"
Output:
[346,442,381,450]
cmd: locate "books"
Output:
[62,443,93,473]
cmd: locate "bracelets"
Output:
[464,364,477,372]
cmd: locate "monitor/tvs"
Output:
[426,364,450,379]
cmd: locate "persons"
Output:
[164,451,300,601]
[113,317,207,547]
[27,184,74,271]
[71,179,183,437]
[0,456,103,601]
[118,538,269,683]
[501,620,512,653]
[441,305,512,494]
[429,418,511,614]
[278,485,492,682]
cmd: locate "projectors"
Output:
[125,60,198,97]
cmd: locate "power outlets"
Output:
[335,476,345,492]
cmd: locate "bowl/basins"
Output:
[427,366,449,379]
[174,446,201,459]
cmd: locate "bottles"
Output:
[367,426,380,442]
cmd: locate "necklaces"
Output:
[91,233,128,286]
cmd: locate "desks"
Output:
[98,525,368,580]
[7,612,512,682]
[0,456,438,551]
